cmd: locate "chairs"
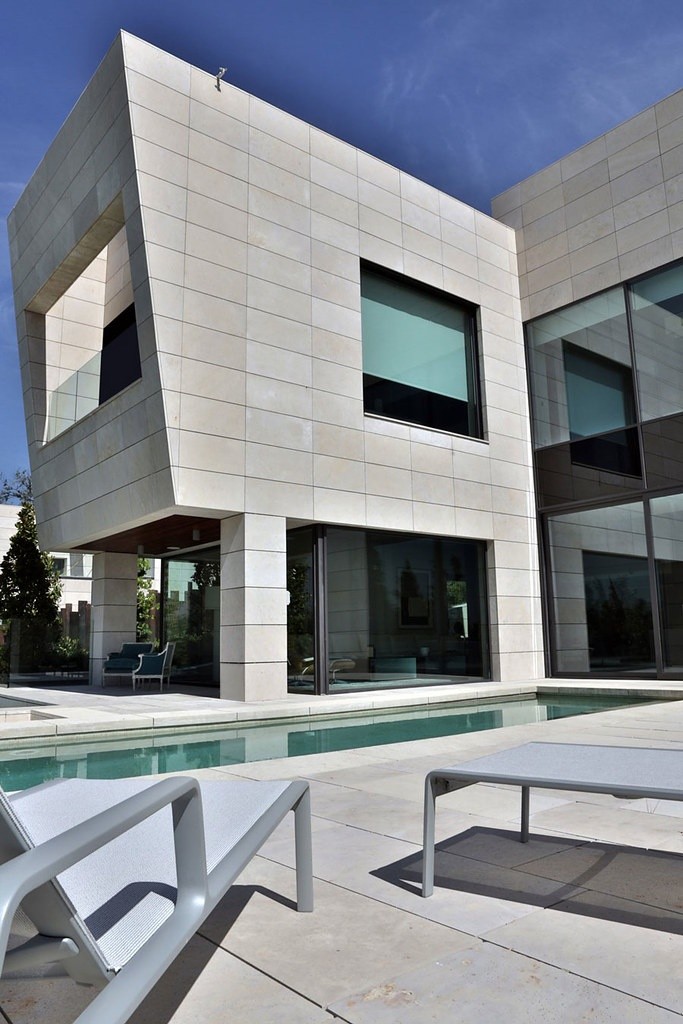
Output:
[423,741,683,899]
[102,643,156,691]
[0,775,314,1024]
[132,641,176,692]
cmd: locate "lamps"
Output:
[138,544,144,556]
[193,530,200,540]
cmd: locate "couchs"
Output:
[286,633,373,684]
